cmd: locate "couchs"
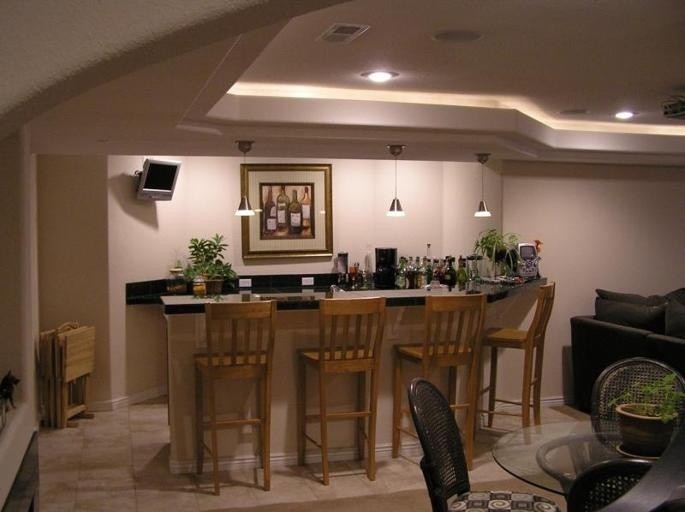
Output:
[568,309,684,423]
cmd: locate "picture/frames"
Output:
[237,161,334,260]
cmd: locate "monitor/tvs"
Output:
[136,158,181,200]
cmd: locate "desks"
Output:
[38,320,97,428]
[151,273,550,479]
[485,418,685,499]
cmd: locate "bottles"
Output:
[331,253,481,295]
[166,268,187,297]
[192,276,207,298]
[260,184,312,236]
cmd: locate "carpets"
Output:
[204,469,570,511]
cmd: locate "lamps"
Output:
[385,145,407,218]
[474,151,493,220]
[235,140,255,218]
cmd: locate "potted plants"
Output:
[175,231,240,301]
[602,365,685,455]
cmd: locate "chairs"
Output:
[472,277,556,446]
[293,294,388,486]
[190,298,279,494]
[391,290,487,474]
[400,375,562,512]
[565,455,669,511]
[589,354,685,449]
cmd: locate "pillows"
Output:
[651,286,685,306]
[663,303,685,338]
[594,283,655,306]
[592,294,670,334]
